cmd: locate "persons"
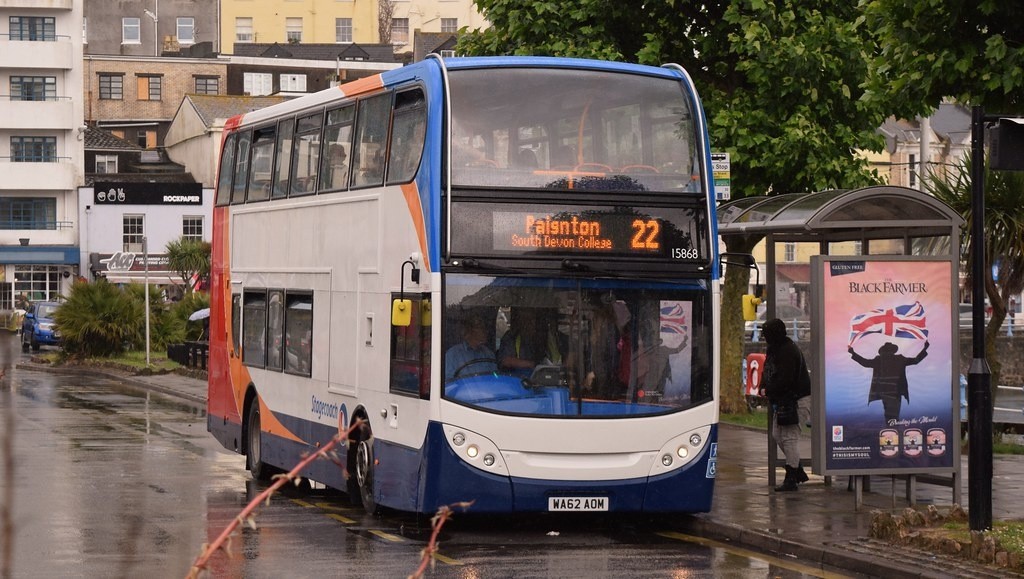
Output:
[520,149,538,169]
[443,309,649,400]
[848,341,929,428]
[761,319,811,490]
[202,317,209,341]
[328,144,348,166]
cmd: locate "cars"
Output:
[960,302,993,329]
[745,299,812,340]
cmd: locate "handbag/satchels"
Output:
[777,399,798,426]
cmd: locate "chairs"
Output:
[261,167,379,197]
[467,158,660,174]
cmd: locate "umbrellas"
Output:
[188,308,210,321]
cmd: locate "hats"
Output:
[879,342,898,354]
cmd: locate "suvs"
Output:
[18,300,65,351]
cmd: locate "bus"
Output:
[206,53,763,518]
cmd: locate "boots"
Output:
[795,461,809,483]
[774,462,797,491]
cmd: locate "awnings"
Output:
[106,274,194,284]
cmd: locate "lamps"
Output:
[77,125,87,141]
[19,238,30,246]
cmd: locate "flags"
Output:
[848,301,928,346]
